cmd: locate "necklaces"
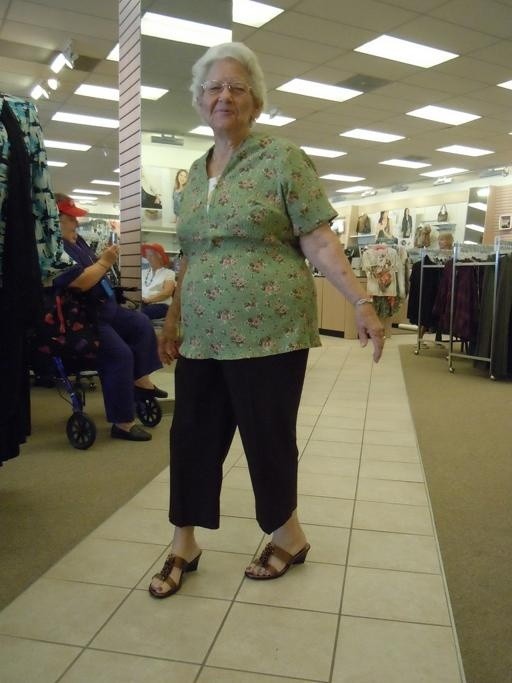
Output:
[144,267,160,286]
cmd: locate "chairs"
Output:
[141,263,175,326]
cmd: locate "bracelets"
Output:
[354,296,377,307]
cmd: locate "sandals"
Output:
[149,549,202,598]
[245,542,311,580]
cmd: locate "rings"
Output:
[381,334,387,341]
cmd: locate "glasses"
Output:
[200,80,251,97]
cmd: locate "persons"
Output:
[48,188,169,441]
[374,209,392,241]
[171,168,189,224]
[399,207,414,239]
[141,240,177,320]
[146,39,388,599]
[438,231,458,250]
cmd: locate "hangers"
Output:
[375,238,398,246]
[423,241,512,261]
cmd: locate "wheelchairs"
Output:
[37,241,162,448]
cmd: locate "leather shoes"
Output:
[135,385,168,398]
[111,423,152,440]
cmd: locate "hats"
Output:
[141,244,169,265]
[53,192,87,216]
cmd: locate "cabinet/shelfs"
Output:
[312,276,408,340]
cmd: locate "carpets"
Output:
[0,359,178,613]
[391,327,432,334]
[398,342,511,682]
